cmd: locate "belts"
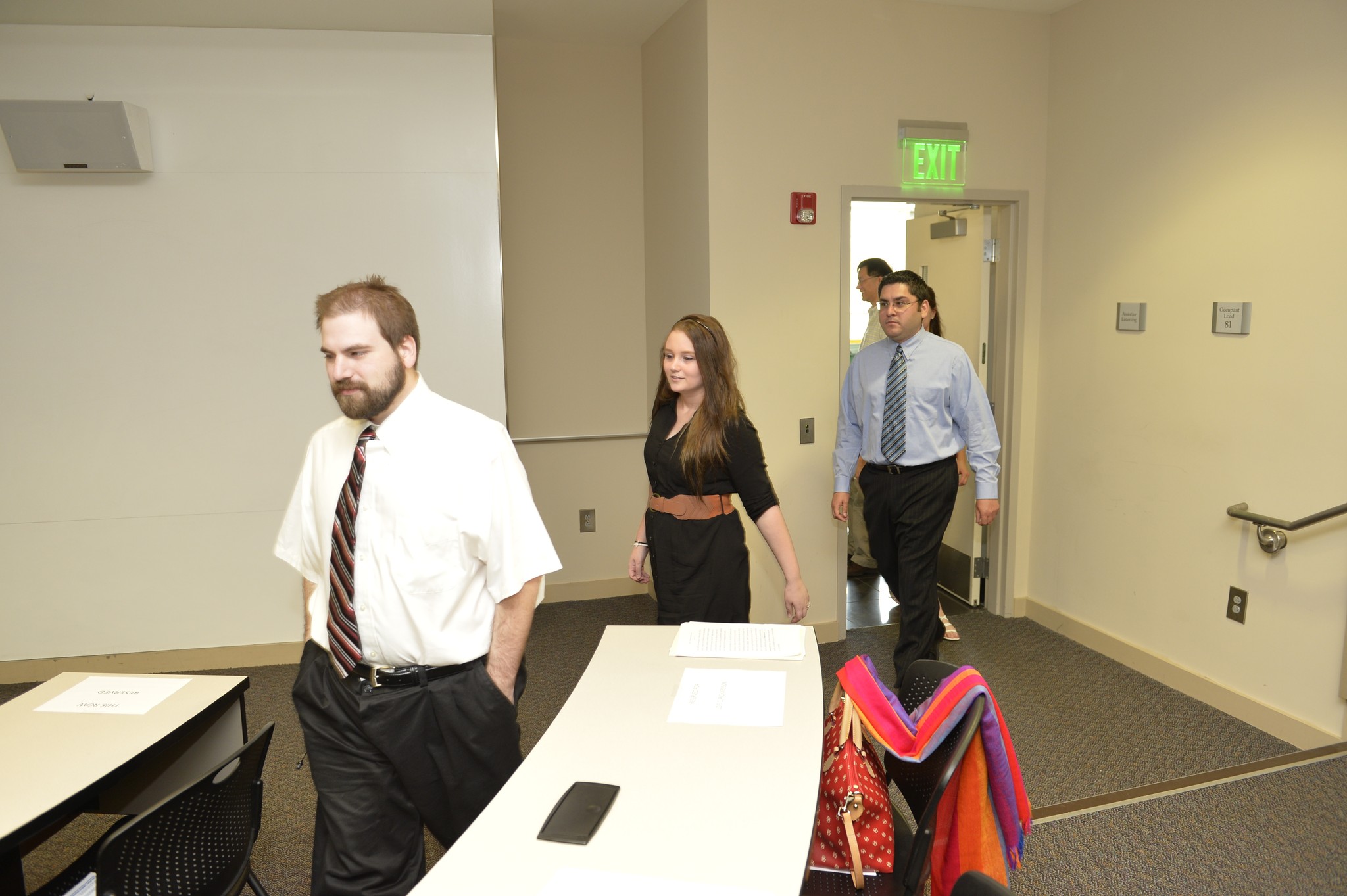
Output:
[646,486,735,520]
[310,639,479,692]
[867,454,954,475]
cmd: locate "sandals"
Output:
[937,614,960,640]
[888,586,901,604]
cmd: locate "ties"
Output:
[325,424,376,681]
[880,344,906,464]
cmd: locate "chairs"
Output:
[800,661,986,896]
[65,720,276,896]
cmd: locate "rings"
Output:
[807,603,812,608]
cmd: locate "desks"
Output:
[0,670,252,896]
[404,624,823,896]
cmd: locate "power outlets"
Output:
[580,509,596,533]
[1226,586,1249,624]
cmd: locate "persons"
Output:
[831,259,1001,689]
[629,314,810,625]
[275,277,566,896]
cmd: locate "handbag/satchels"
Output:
[810,680,896,889]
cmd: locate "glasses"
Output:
[876,299,921,311]
[857,275,880,285]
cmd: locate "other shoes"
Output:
[847,560,880,577]
[847,553,853,560]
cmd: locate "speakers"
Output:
[1,96,156,172]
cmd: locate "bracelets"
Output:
[634,541,648,548]
